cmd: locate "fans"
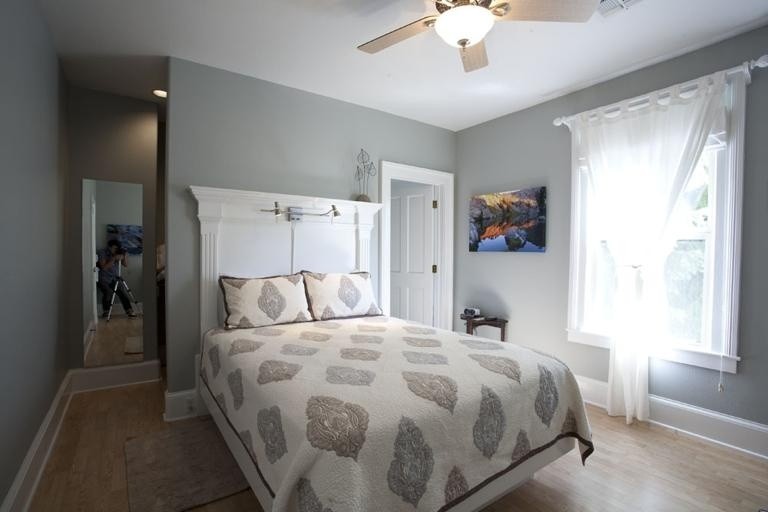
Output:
[357,0,602,73]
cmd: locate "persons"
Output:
[96,238,138,320]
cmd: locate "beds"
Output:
[186,184,576,512]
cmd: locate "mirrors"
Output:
[82,178,144,368]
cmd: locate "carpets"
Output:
[124,336,143,354]
[124,418,250,511]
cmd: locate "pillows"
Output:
[218,270,384,330]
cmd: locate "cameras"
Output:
[116,247,126,254]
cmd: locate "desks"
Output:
[467,316,508,341]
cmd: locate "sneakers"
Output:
[128,313,138,319]
[103,312,111,318]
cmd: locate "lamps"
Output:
[435,5,495,53]
[260,202,342,217]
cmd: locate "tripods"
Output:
[106,255,144,322]
